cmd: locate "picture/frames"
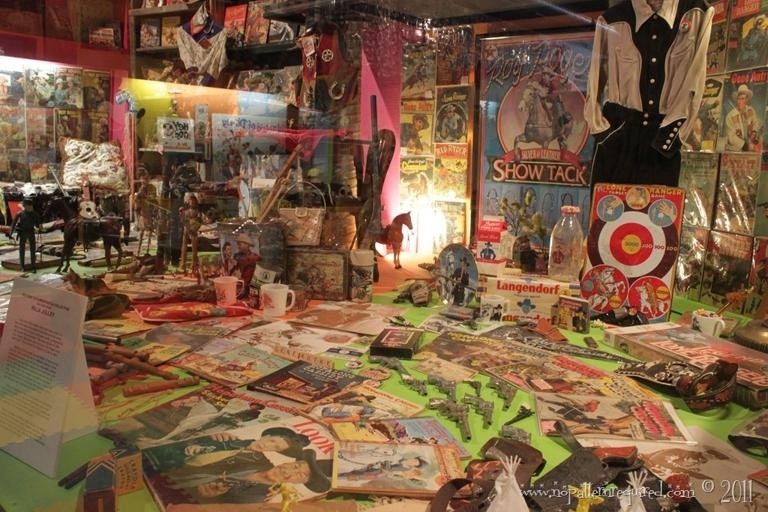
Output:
[473,22,622,254]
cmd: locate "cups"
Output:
[481,294,510,324]
[690,310,726,338]
[260,283,296,315]
[213,276,245,307]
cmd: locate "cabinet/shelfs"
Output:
[127,0,333,220]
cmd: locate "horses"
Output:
[511,79,576,164]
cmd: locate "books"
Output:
[0,2,766,510]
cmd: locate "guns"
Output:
[498,380,517,409]
[504,405,534,424]
[502,426,531,446]
[429,398,471,441]
[464,393,494,424]
[463,381,481,397]
[402,379,427,396]
[368,356,410,375]
[428,375,456,403]
[584,447,637,472]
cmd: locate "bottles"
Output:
[350,248,375,303]
[548,206,583,281]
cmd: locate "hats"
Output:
[235,233,255,249]
[295,447,336,496]
[360,392,378,404]
[731,84,754,101]
[752,14,765,27]
[411,112,430,129]
[248,401,267,412]
[459,255,470,268]
[575,306,586,314]
[260,425,311,460]
[443,104,456,112]
[415,455,428,470]
[494,303,503,311]
[538,66,558,77]
[517,297,536,310]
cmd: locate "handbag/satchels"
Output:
[276,181,327,248]
